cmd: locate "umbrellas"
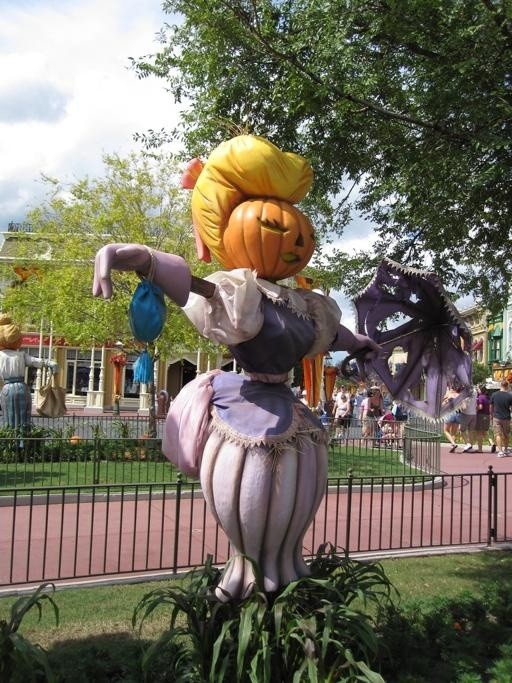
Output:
[338,257,477,425]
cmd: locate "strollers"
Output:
[371,410,405,450]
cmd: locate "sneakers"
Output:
[450,443,512,458]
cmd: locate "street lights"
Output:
[113,339,126,416]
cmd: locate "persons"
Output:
[87,131,383,609]
[1,314,57,447]
[291,381,511,458]
